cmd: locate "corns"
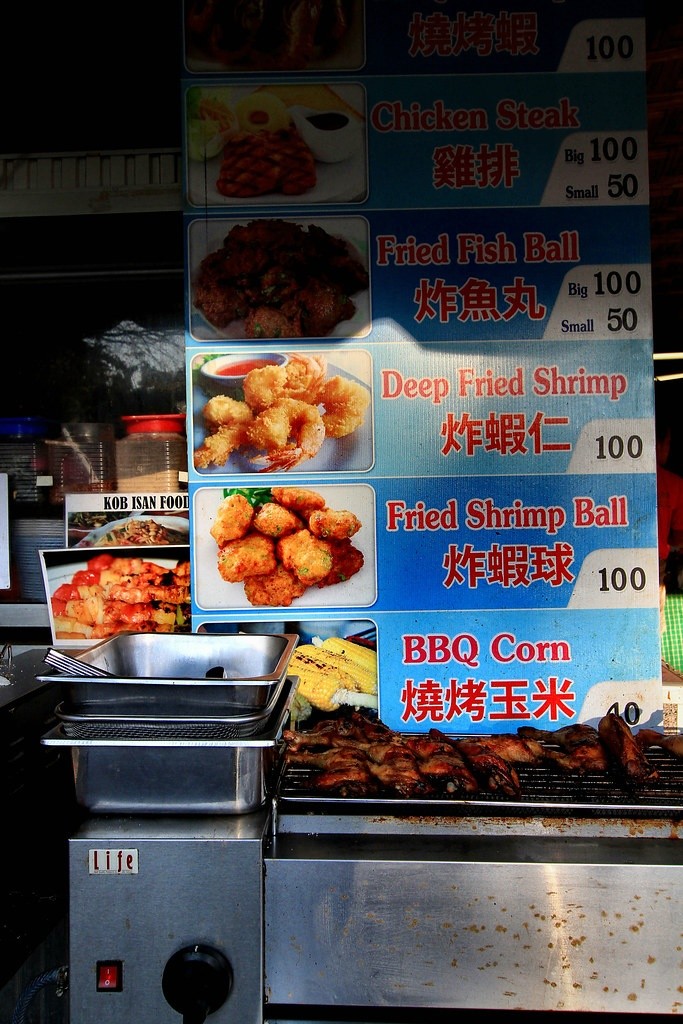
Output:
[284,637,379,710]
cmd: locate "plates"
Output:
[190,152,366,203]
[193,362,373,472]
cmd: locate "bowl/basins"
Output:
[200,354,289,386]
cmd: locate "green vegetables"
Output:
[222,488,275,510]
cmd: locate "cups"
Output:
[287,104,360,164]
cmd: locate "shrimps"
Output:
[193,351,372,473]
